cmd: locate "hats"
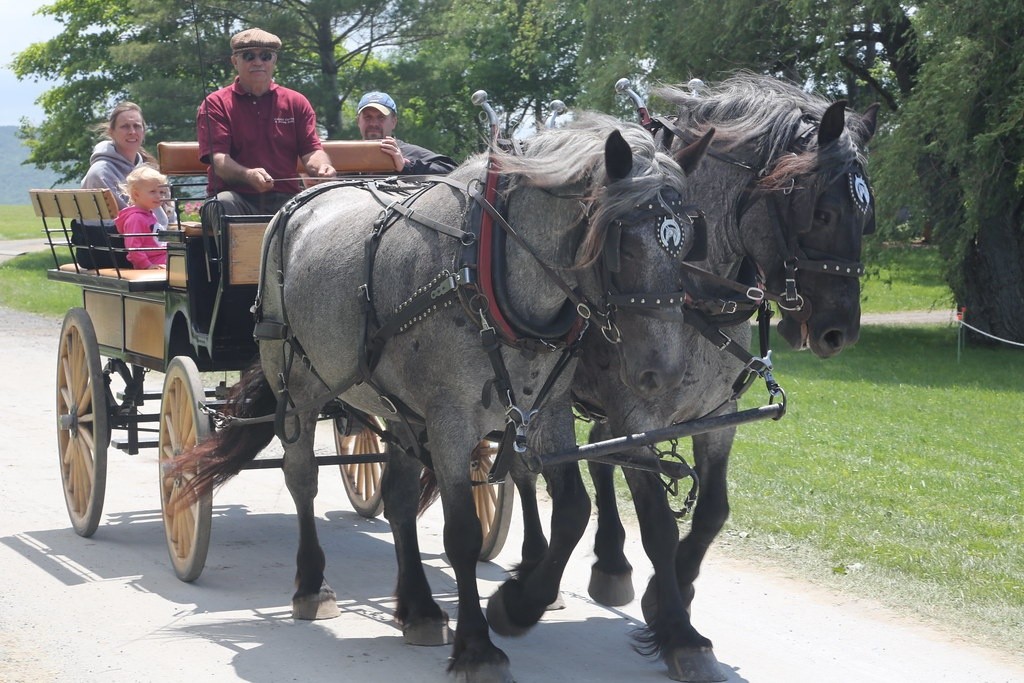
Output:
[230,28,282,54]
[357,92,397,116]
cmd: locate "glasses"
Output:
[235,50,275,62]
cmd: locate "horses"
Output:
[151,70,882,683]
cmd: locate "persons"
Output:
[115,163,171,271]
[196,28,339,356]
[70,101,178,270]
[332,91,460,180]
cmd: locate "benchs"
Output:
[31,188,166,293]
[157,140,397,236]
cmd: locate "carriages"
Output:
[27,67,886,682]
